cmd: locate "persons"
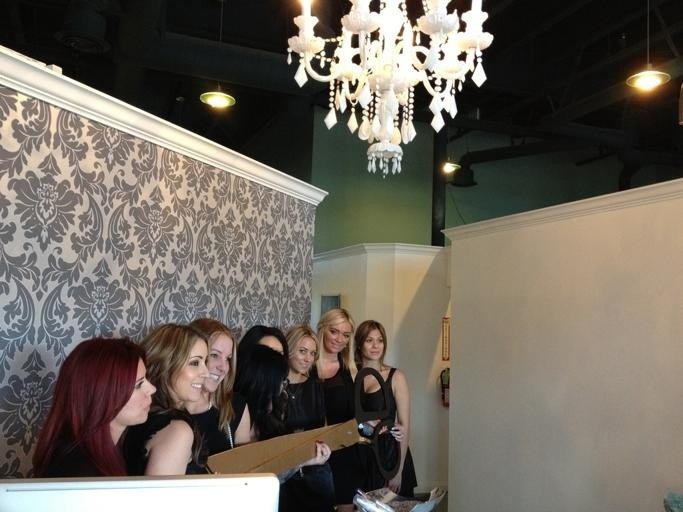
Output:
[31,307,417,512]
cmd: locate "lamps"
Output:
[283,0,498,181]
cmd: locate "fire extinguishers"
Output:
[440,367,450,408]
